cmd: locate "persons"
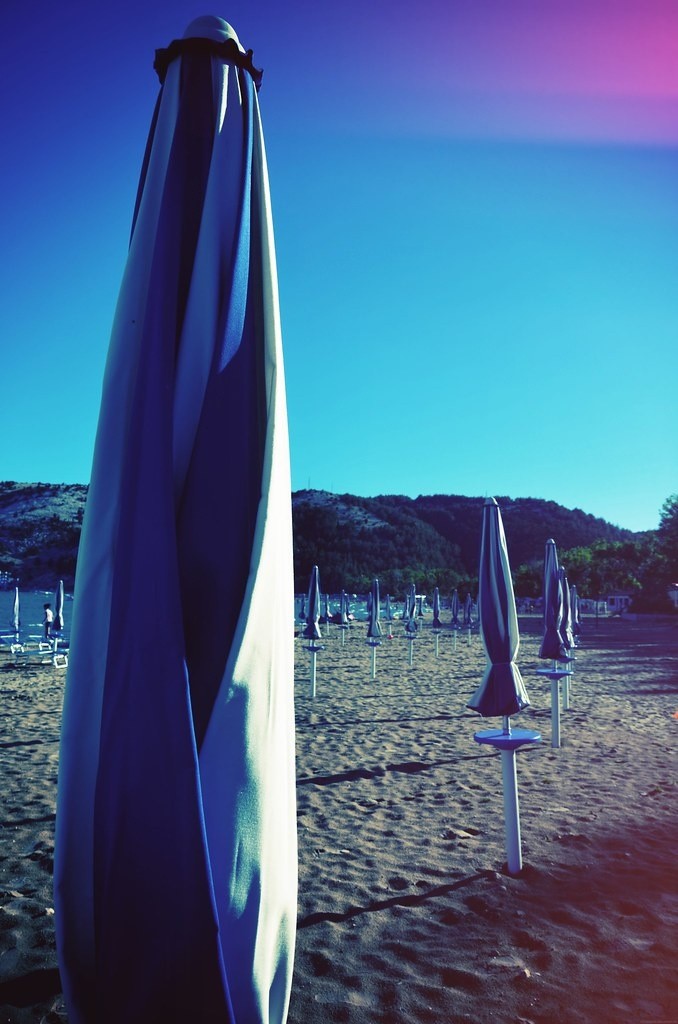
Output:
[41,603,54,640]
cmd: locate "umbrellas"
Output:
[366,579,381,679]
[417,595,425,632]
[401,584,419,665]
[11,586,23,642]
[50,18,300,1024]
[384,594,393,635]
[448,589,460,651]
[340,590,350,646]
[53,580,64,655]
[302,566,323,696]
[429,587,443,658]
[537,537,583,747]
[299,593,307,619]
[465,498,540,873]
[464,592,474,644]
[324,593,333,633]
